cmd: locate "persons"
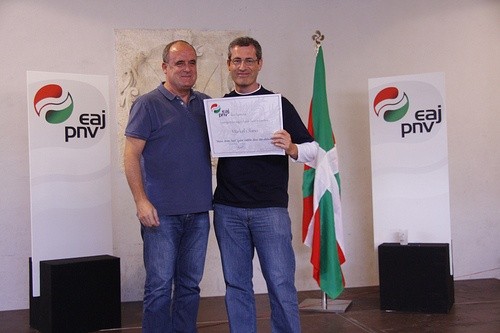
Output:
[124,40,213,333]
[213,37,319,333]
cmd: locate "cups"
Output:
[398,231,408,246]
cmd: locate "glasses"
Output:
[229,58,258,66]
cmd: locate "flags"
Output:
[302,46,346,301]
